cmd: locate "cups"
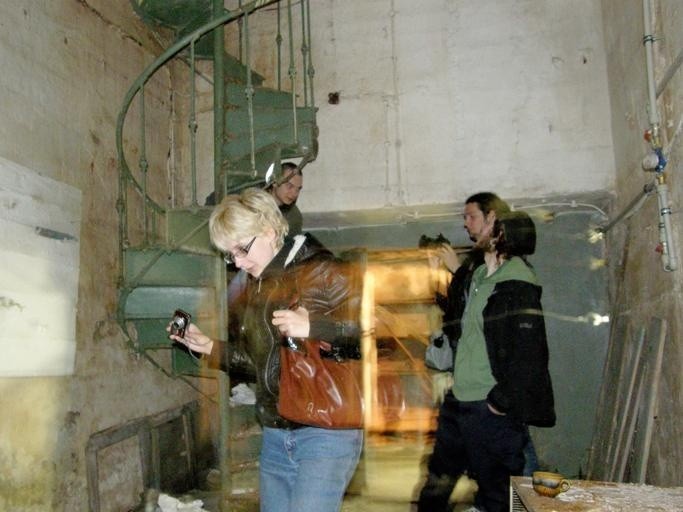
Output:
[532,471,570,497]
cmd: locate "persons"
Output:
[418,211,555,512]
[229,162,304,405]
[428,192,539,512]
[166,187,364,512]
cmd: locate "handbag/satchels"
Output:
[424,335,455,371]
[276,344,406,431]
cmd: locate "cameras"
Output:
[168,307,192,346]
[418,233,451,254]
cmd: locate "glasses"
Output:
[223,236,256,264]
